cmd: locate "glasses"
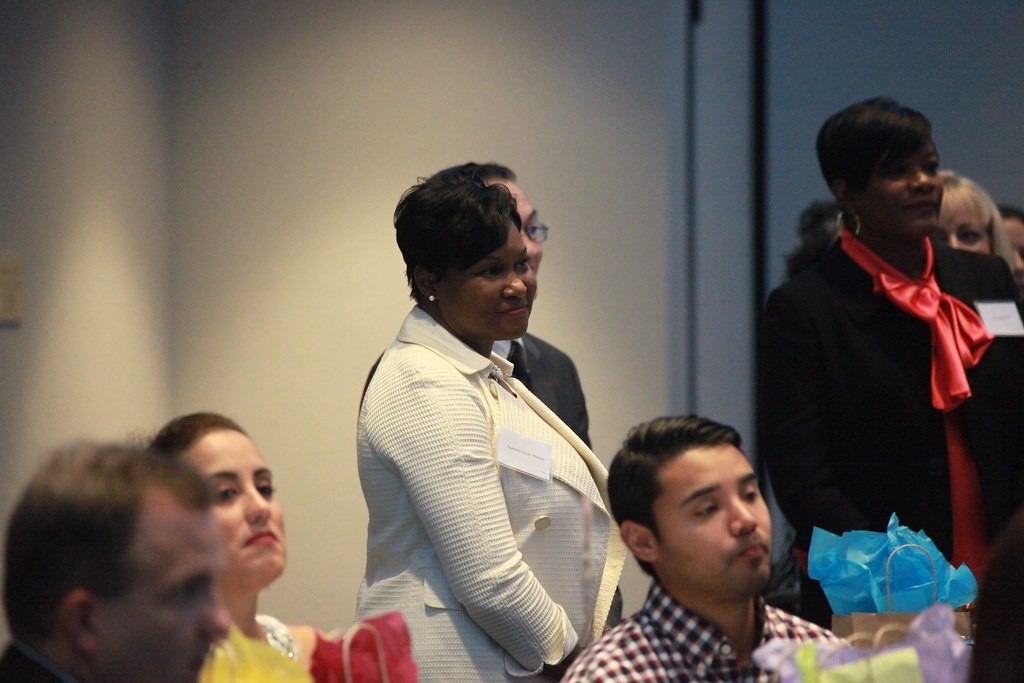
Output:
[519,221,550,243]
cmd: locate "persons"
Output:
[358,162,623,637]
[145,412,320,683]
[562,414,840,683]
[0,438,231,683]
[756,98,1024,683]
[936,169,1024,291]
[785,201,840,277]
[357,179,627,683]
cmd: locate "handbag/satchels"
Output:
[831,545,973,683]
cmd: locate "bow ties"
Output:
[841,224,995,413]
